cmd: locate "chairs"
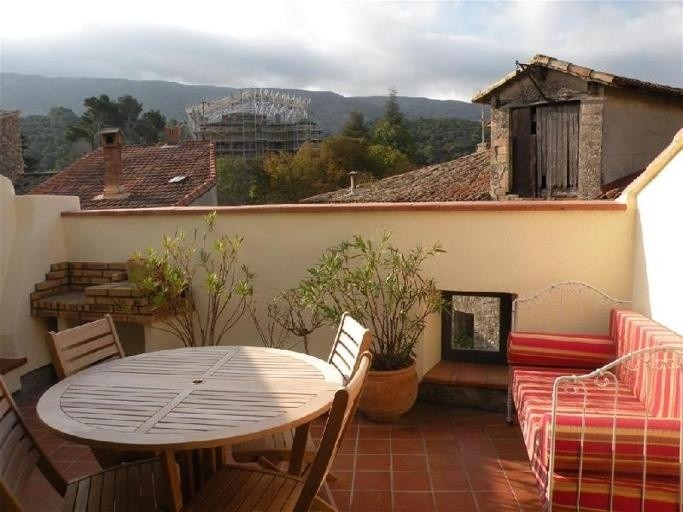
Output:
[232,311,372,511]
[181,351,373,512]
[44,314,209,495]
[0,377,184,511]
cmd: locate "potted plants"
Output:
[295,229,452,424]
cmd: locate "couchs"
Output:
[506,308,682,510]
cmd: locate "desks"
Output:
[35,347,343,510]
